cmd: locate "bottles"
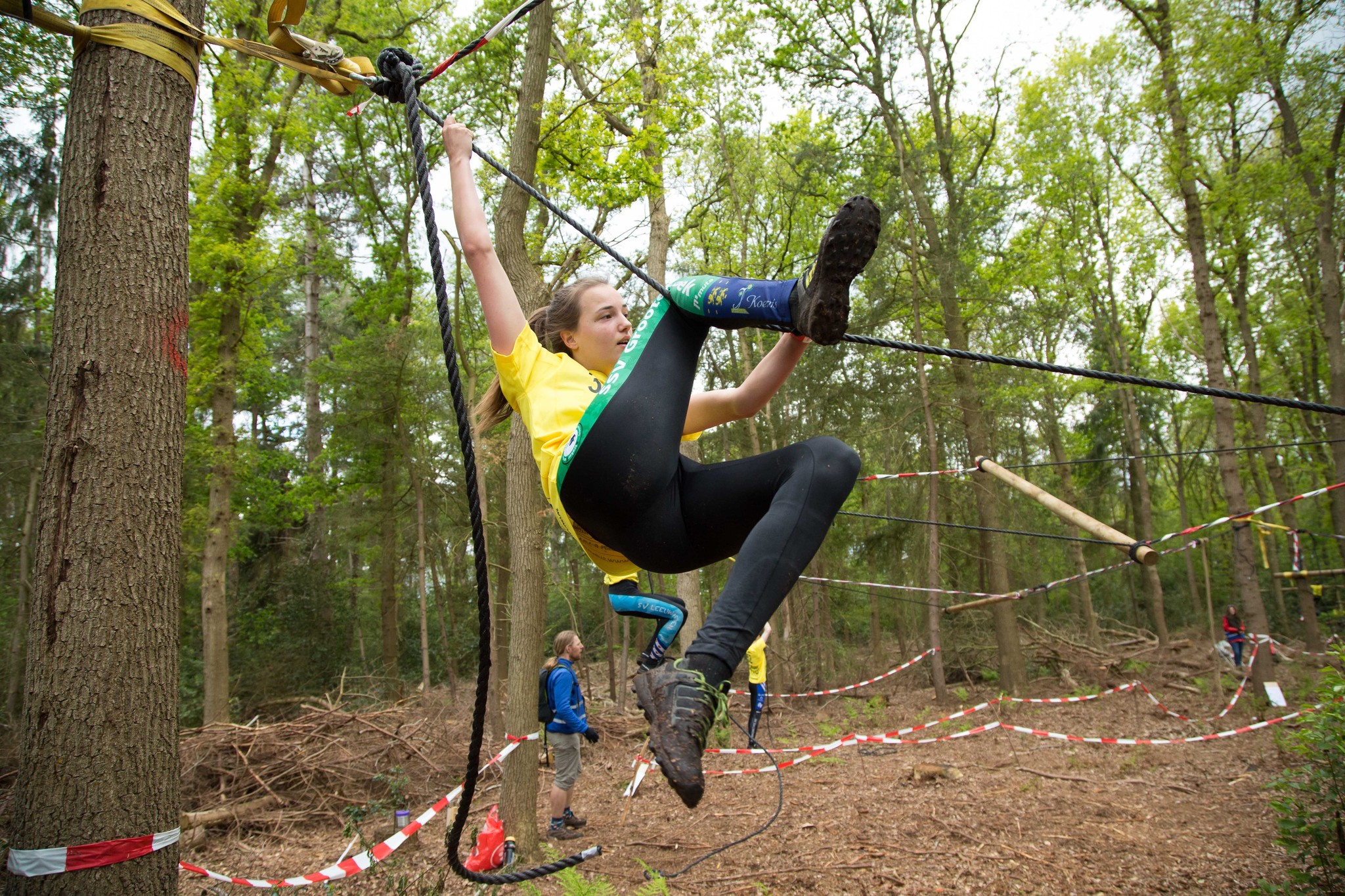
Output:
[395,810,410,828]
[503,836,516,867]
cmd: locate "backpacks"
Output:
[538,665,570,723]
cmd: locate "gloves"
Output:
[583,727,600,743]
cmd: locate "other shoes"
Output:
[637,652,648,665]
[547,824,576,839]
[637,662,655,674]
[563,811,586,827]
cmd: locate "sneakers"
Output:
[632,658,733,809]
[796,194,881,346]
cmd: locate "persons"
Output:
[745,621,772,749]
[542,630,600,840]
[1223,605,1246,671]
[441,122,881,809]
[603,572,689,675]
